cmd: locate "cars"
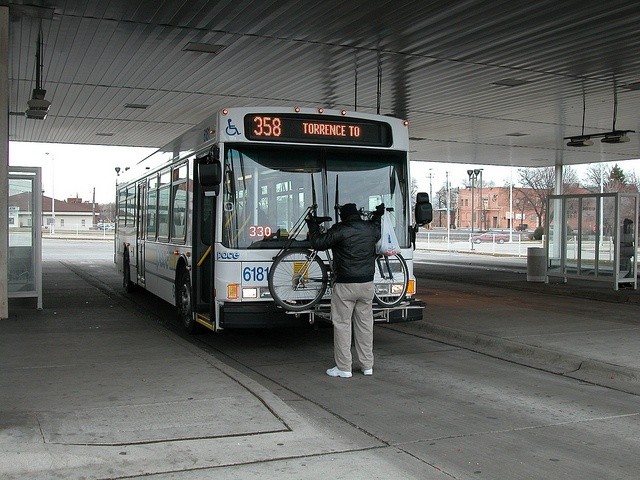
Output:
[472,231,510,244]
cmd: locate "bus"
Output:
[114,107,432,333]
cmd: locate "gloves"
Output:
[376,203,385,216]
[305,213,316,227]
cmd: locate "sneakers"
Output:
[362,367,373,375]
[326,367,352,377]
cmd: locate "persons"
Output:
[309,201,384,377]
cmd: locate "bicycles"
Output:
[268,208,409,310]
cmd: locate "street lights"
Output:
[467,169,481,250]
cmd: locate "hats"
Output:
[338,203,361,214]
